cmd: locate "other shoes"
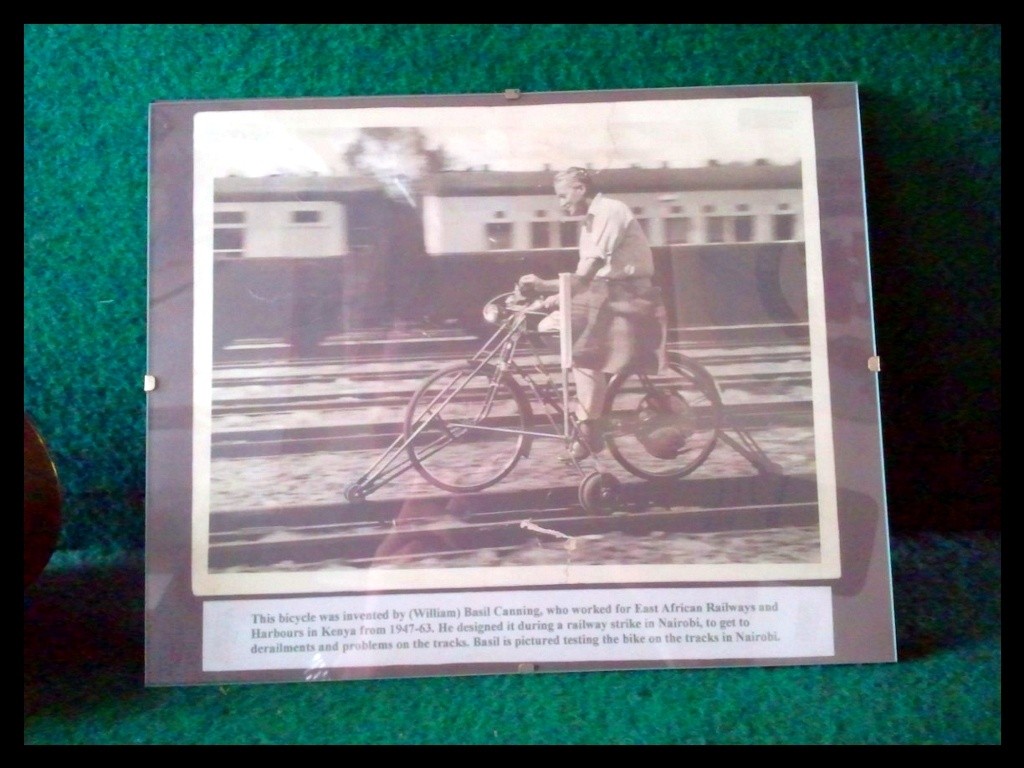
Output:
[555,436,605,461]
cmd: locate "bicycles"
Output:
[345,280,784,516]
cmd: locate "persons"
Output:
[519,167,663,462]
